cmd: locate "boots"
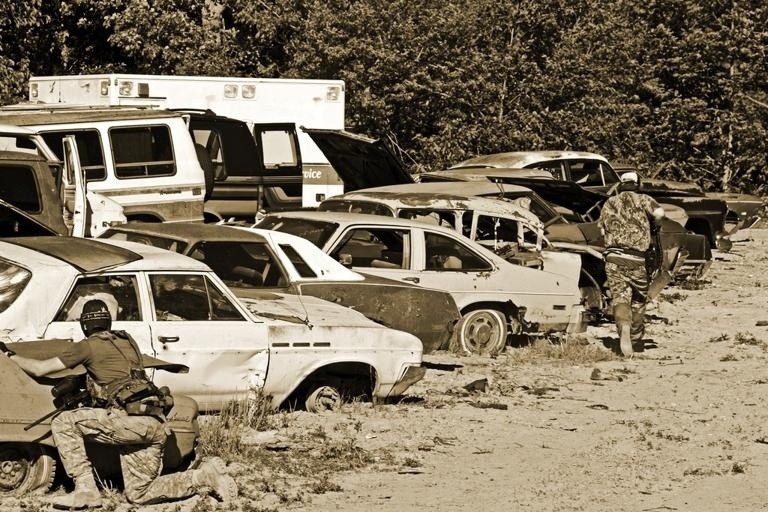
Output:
[52,472,102,508]
[192,456,238,502]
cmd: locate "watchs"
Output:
[7,352,16,357]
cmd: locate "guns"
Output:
[25,376,89,431]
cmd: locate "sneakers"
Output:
[619,324,632,356]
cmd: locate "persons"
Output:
[596,171,666,357]
[0,298,239,509]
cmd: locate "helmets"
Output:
[79,299,112,338]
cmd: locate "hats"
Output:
[620,171,638,183]
